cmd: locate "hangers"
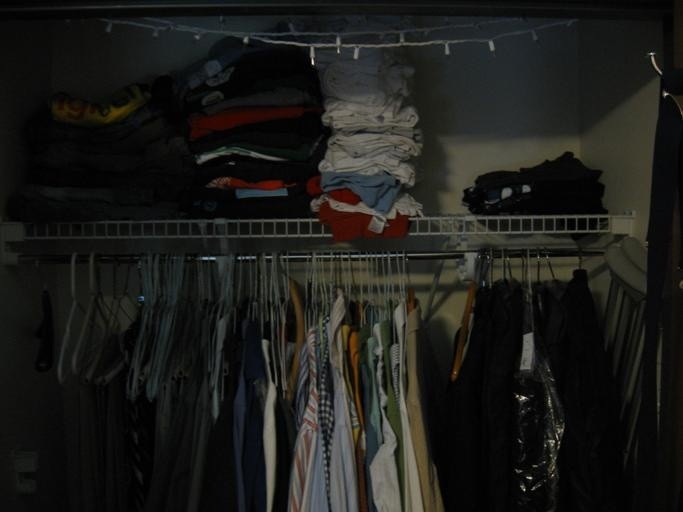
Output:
[41,251,415,401]
[449,247,582,381]
